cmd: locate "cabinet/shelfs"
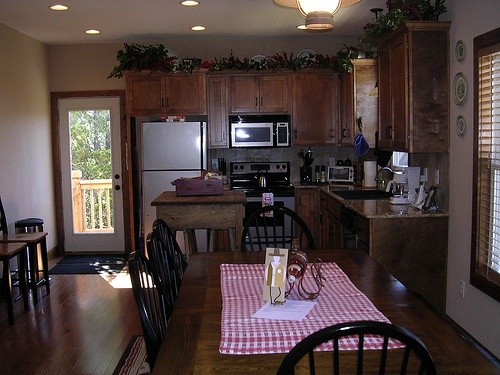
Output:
[293,73,336,146]
[121,70,207,116]
[208,74,229,150]
[228,73,291,114]
[338,64,377,148]
[295,188,368,251]
[378,28,449,152]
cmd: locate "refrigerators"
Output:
[140,121,210,260]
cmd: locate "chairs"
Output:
[277,320,437,375]
[0,197,51,326]
[127,218,188,351]
[240,204,315,253]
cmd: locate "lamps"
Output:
[274,0,364,29]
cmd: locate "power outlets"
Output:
[459,281,464,297]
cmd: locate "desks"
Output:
[152,248,500,375]
[151,191,247,251]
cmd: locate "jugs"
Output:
[386,180,409,197]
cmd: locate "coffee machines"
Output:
[384,166,422,204]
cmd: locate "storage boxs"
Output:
[171,178,224,196]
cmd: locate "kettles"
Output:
[257,168,268,187]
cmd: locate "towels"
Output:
[260,193,273,217]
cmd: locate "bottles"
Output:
[287,250,308,282]
[315,165,325,184]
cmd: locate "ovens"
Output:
[328,166,354,183]
[243,189,297,250]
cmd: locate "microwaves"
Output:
[229,114,292,149]
[231,161,294,189]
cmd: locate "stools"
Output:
[14,218,46,285]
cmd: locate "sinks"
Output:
[332,190,394,199]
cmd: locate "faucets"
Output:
[376,167,406,196]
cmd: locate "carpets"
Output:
[48,255,126,275]
[111,334,151,375]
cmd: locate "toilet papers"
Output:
[364,161,376,186]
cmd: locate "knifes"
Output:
[218,157,226,176]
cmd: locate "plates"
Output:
[162,49,320,70]
[455,40,466,62]
[452,72,468,106]
[455,114,470,137]
